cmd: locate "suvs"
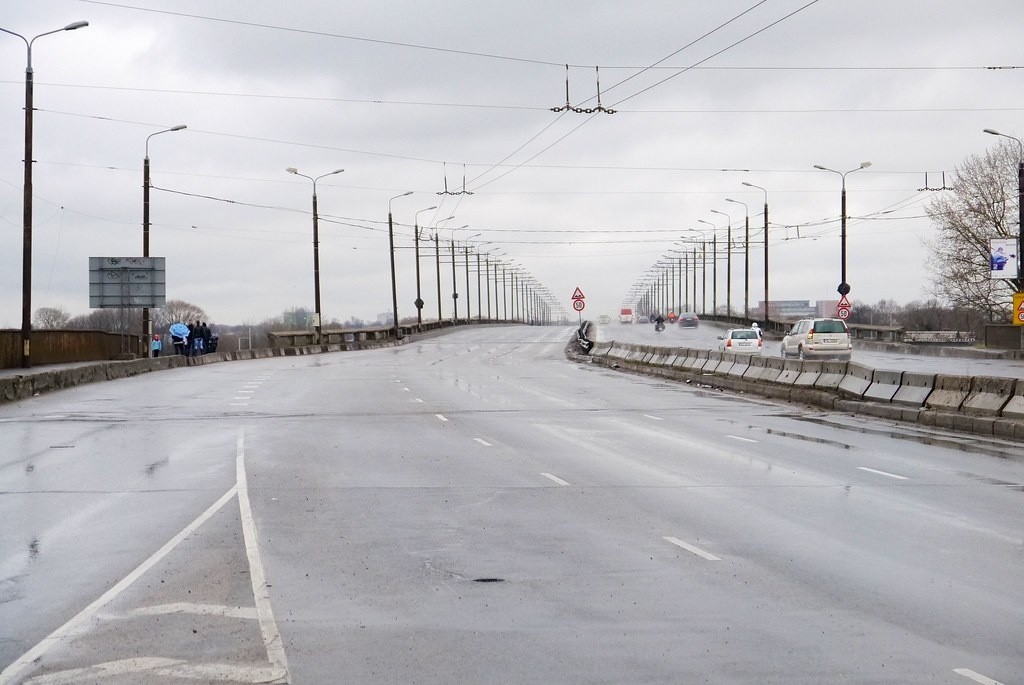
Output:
[781,317,854,363]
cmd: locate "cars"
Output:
[599,315,610,324]
[637,316,649,323]
[717,328,763,357]
[678,312,700,329]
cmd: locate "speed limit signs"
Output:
[838,308,850,319]
[573,300,585,311]
[1018,312,1024,321]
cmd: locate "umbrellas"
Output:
[169,323,190,339]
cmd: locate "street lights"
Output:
[0,21,90,365]
[622,234,699,315]
[710,210,731,323]
[387,189,414,340]
[486,248,570,326]
[285,167,344,344]
[141,124,191,354]
[412,206,437,332]
[465,234,481,325]
[434,216,454,328]
[813,162,874,320]
[742,182,770,331]
[478,241,492,324]
[689,228,706,321]
[450,225,468,326]
[982,128,1023,293]
[727,198,749,325]
[698,220,716,322]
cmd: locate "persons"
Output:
[178,320,212,357]
[151,334,162,358]
[637,310,677,324]
[654,315,666,331]
[750,322,763,341]
[171,334,185,356]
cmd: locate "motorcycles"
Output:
[650,316,656,323]
[655,320,666,333]
[668,316,676,324]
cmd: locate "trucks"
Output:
[619,308,634,324]
